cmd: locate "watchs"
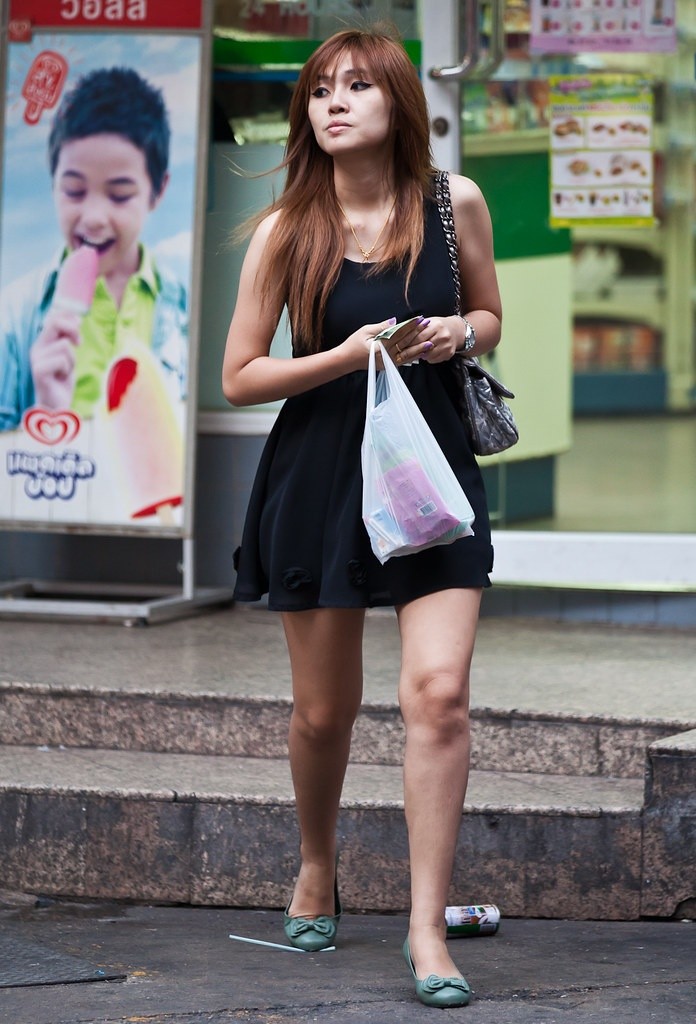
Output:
[456,316,476,354]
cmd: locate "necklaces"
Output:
[335,188,398,260]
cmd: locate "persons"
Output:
[1,68,188,434]
[221,29,502,1008]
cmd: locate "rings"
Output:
[397,354,401,363]
[395,344,402,352]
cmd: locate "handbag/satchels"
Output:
[437,170,518,455]
[360,339,475,565]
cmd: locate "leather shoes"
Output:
[402,935,471,1008]
[285,848,342,953]
